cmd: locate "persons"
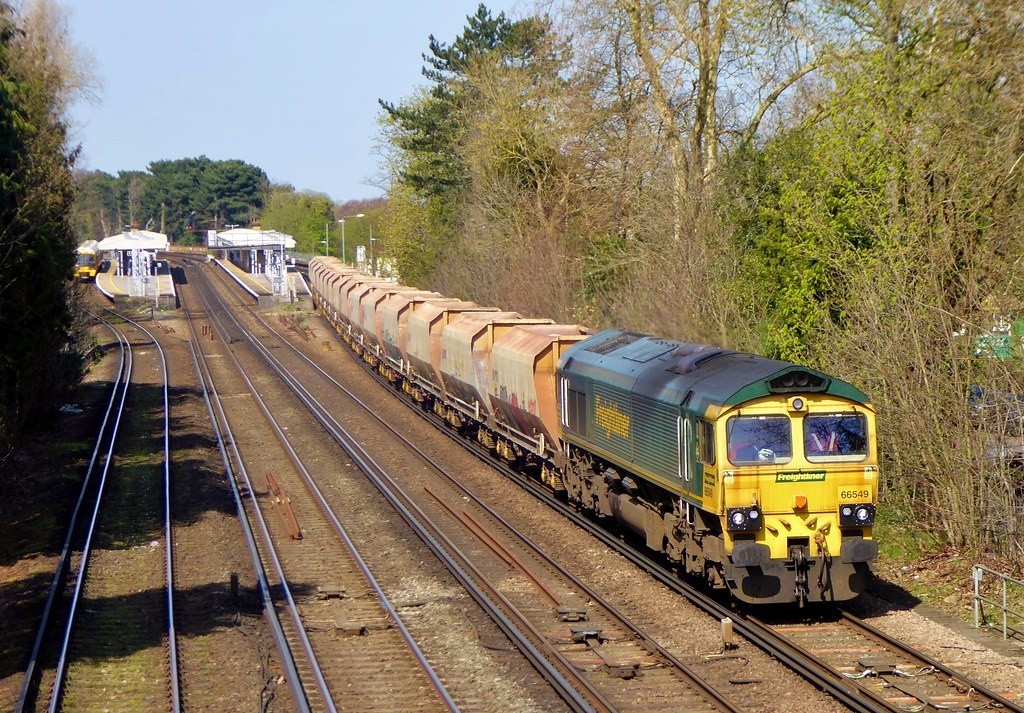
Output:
[727,422,767,461]
[803,417,850,456]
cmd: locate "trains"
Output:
[308,255,880,608]
[71,239,103,283]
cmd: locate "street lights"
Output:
[342,214,365,264]
[326,220,345,256]
